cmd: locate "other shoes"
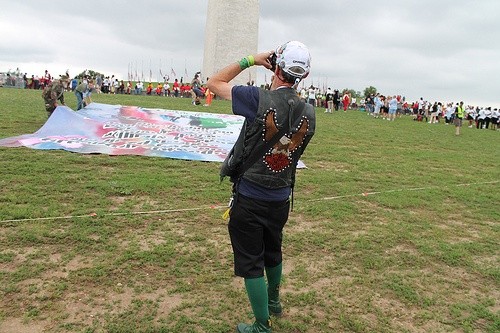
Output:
[413,119,500,131]
[203,104,209,106]
[236,322,273,333]
[367,113,395,121]
[268,299,282,316]
[324,109,334,114]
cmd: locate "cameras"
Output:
[270,54,277,72]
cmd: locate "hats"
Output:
[275,40,312,78]
[59,75,69,82]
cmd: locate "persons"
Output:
[42,74,70,118]
[247,80,499,135]
[207,40,317,332]
[74,79,94,111]
[0,68,216,106]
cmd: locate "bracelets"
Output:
[237,55,255,69]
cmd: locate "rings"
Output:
[269,51,272,55]
